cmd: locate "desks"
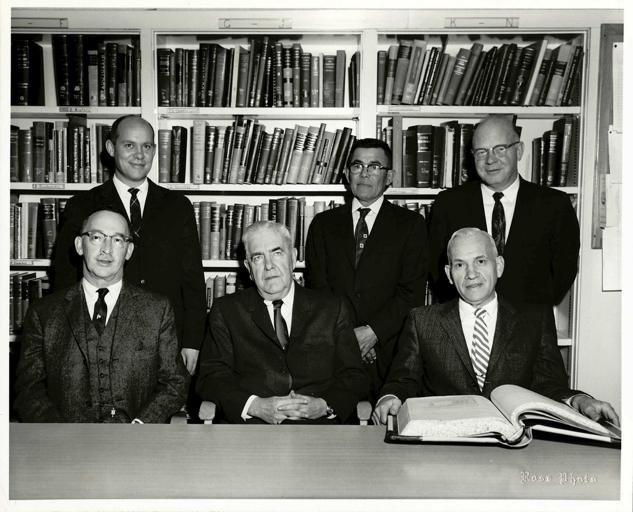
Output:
[10,422,620,499]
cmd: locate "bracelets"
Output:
[326,402,334,417]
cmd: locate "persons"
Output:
[47,115,206,376]
[427,114,579,316]
[15,209,190,424]
[184,220,365,424]
[304,139,429,385]
[368,228,620,429]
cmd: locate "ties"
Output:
[93,289,108,337]
[492,193,506,257]
[272,300,290,350]
[355,208,372,269]
[128,188,143,241]
[472,310,490,393]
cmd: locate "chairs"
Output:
[196,401,372,425]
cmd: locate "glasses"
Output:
[80,231,131,247]
[472,142,521,158]
[348,163,390,175]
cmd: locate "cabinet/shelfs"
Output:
[8,7,597,422]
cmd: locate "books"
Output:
[425,31,586,190]
[10,120,117,183]
[157,35,360,107]
[160,120,357,184]
[10,194,68,259]
[9,273,50,335]
[383,383,620,450]
[190,197,343,311]
[11,33,140,107]
[376,40,432,217]
